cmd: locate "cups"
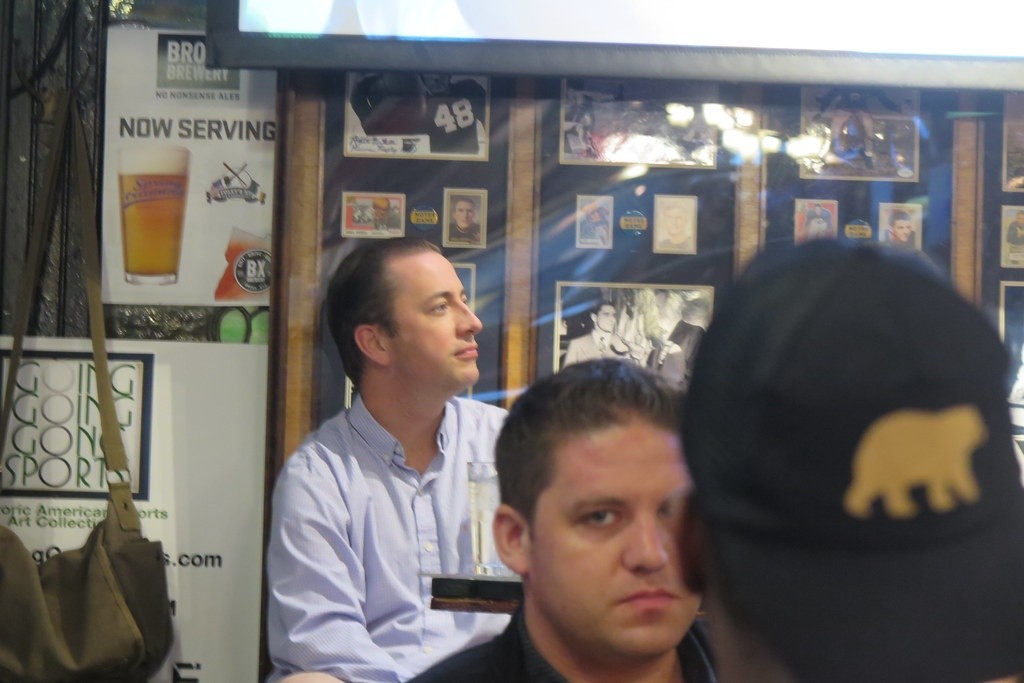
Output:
[117,147,190,286]
[212,226,270,302]
[466,461,515,578]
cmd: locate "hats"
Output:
[685,239,1024,683]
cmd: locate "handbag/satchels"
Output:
[0,483,173,683]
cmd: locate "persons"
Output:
[885,209,915,245]
[449,197,481,242]
[373,198,401,230]
[563,288,706,382]
[407,358,720,683]
[1006,211,1024,261]
[659,207,691,249]
[672,235,1024,683]
[265,238,521,682]
